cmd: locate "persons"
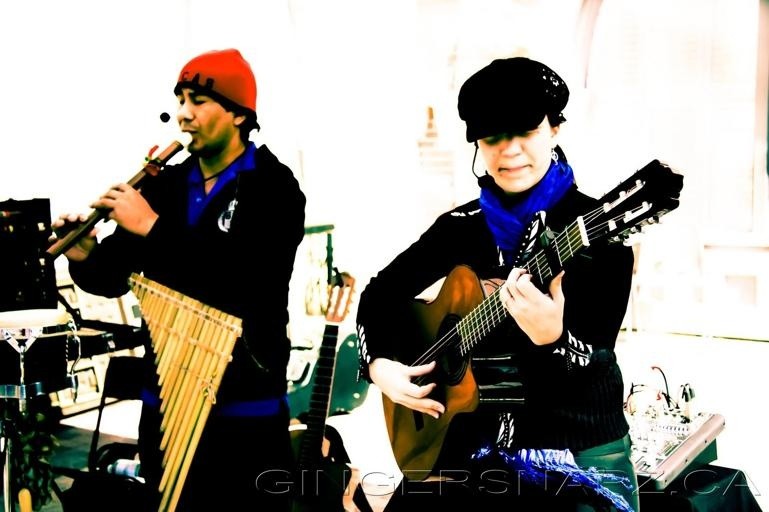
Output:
[51,49,307,512]
[355,57,640,511]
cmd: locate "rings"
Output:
[502,298,510,308]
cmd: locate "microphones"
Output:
[472,145,494,188]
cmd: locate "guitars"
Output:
[381,159,684,482]
[256,272,361,511]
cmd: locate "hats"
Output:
[174,48,257,121]
[456,55,569,143]
